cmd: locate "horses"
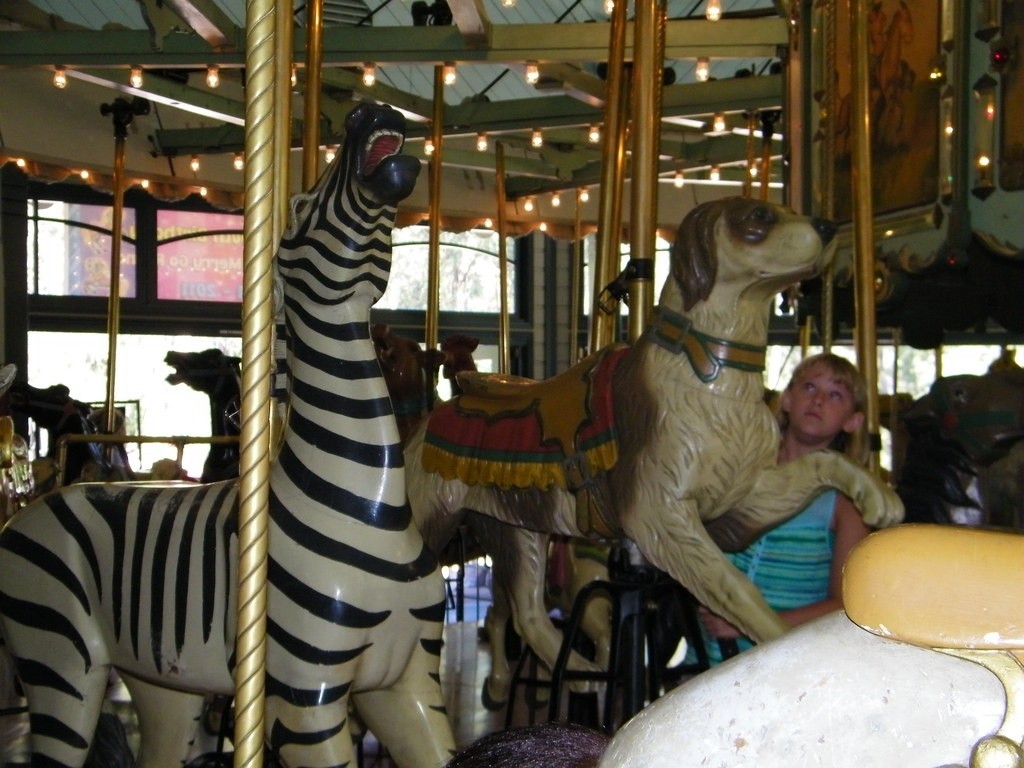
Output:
[1,324,575,766]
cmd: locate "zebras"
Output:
[3,99,447,766]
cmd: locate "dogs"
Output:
[403,196,906,711]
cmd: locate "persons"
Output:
[694,352,863,675]
